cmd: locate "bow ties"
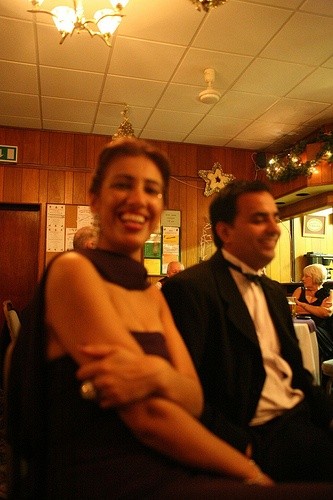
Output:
[223,260,273,289]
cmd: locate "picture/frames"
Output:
[303,214,327,237]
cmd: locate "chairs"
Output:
[3,301,21,382]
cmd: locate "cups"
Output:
[286,297,297,319]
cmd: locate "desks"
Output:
[293,316,320,386]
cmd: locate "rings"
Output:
[81,381,97,399]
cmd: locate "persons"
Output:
[158,260,185,287]
[291,264,333,359]
[72,226,99,250]
[162,180,333,485]
[9,139,273,486]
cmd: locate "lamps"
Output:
[26,0,130,47]
